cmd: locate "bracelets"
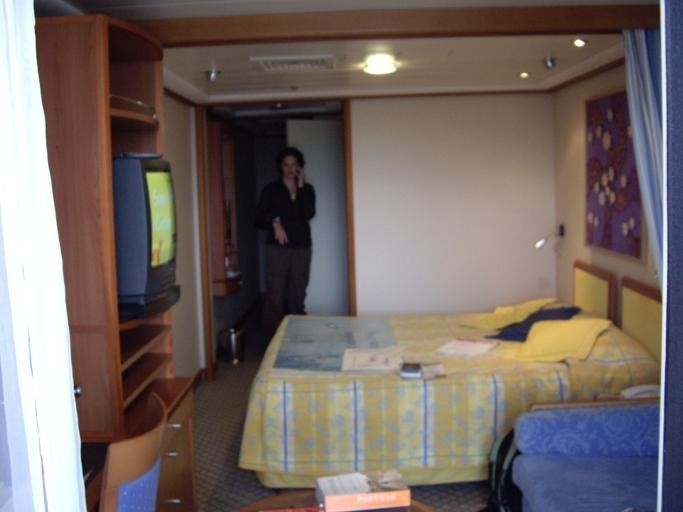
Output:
[274,220,280,223]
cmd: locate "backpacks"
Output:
[489,428,523,512]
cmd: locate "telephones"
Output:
[620,382,660,401]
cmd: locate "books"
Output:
[314,468,412,512]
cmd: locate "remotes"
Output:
[123,150,162,158]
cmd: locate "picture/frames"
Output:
[585,91,648,259]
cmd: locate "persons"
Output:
[252,145,316,362]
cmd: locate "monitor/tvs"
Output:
[114,158,178,305]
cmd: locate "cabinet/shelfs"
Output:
[35,15,199,511]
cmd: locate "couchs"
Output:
[491,399,666,510]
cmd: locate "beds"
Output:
[254,260,662,489]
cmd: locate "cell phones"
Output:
[400,362,420,379]
